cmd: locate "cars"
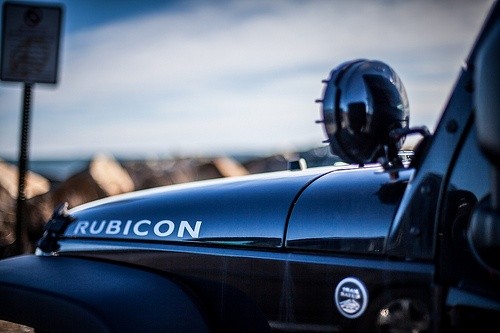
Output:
[0,0,500,333]
